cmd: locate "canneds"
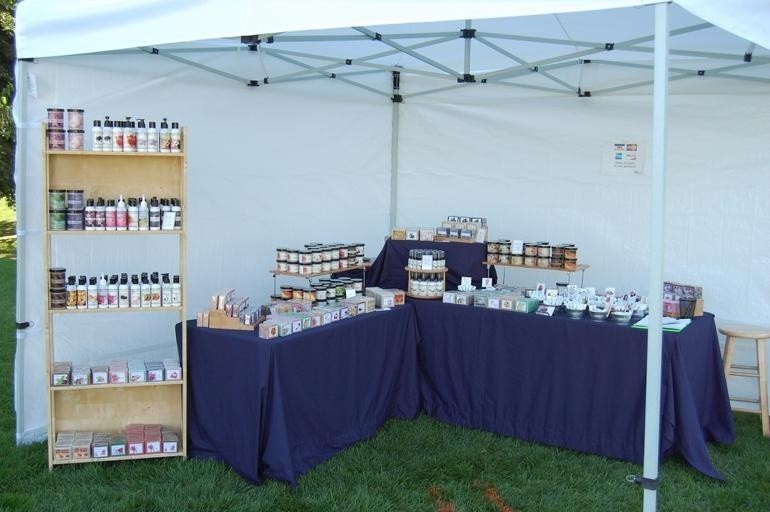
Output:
[276,242,370,307]
[487,239,577,271]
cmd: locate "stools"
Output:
[718,322,770,438]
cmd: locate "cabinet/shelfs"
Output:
[38,120,189,472]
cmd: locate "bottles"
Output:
[51,266,181,309]
[406,249,447,297]
[48,188,181,230]
[47,108,183,154]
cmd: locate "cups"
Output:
[680,298,698,318]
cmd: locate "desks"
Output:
[404,294,737,481]
[371,236,498,293]
[174,302,421,486]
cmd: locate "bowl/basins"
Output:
[543,299,646,324]
